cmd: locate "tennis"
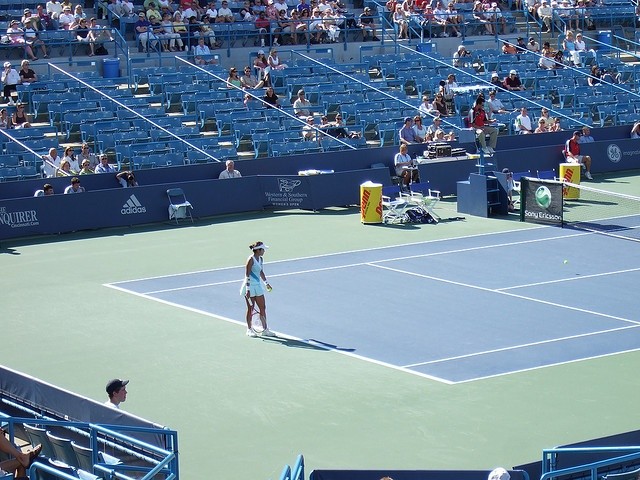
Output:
[563,259,568,264]
[267,288,271,292]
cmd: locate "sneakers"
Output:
[409,181,415,185]
[457,32,462,38]
[547,29,550,32]
[481,147,490,154]
[584,171,592,180]
[363,37,367,41]
[8,101,14,105]
[245,328,257,337]
[576,28,582,32]
[81,39,83,42]
[488,148,495,153]
[31,42,34,47]
[401,180,406,187]
[43,53,48,58]
[571,28,574,31]
[501,31,505,34]
[372,36,380,40]
[261,329,275,336]
[490,32,495,35]
[443,33,449,37]
[32,57,39,61]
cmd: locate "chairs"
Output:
[380,183,409,226]
[507,170,536,212]
[267,0,640,158]
[536,169,557,182]
[164,188,194,226]
[409,180,441,224]
[0,2,267,180]
[22,419,108,473]
[561,148,586,179]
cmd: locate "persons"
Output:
[539,50,555,69]
[533,119,547,133]
[488,73,505,92]
[488,91,510,113]
[564,131,594,180]
[240,241,275,337]
[12,103,32,129]
[0,61,21,105]
[439,74,458,112]
[526,37,539,52]
[227,67,243,90]
[552,51,565,69]
[577,127,594,143]
[447,132,457,141]
[61,146,81,173]
[267,48,289,70]
[427,117,446,141]
[116,170,139,188]
[330,114,346,128]
[219,160,242,179]
[262,88,281,109]
[540,42,556,58]
[575,0,594,30]
[452,45,470,67]
[468,97,499,154]
[538,107,554,131]
[501,168,514,211]
[293,89,315,120]
[563,31,576,57]
[432,129,446,142]
[242,92,252,112]
[393,144,419,188]
[19,60,37,84]
[398,117,421,146]
[254,50,271,81]
[239,66,272,90]
[473,92,485,110]
[412,115,427,143]
[422,133,433,144]
[432,93,450,117]
[503,70,526,91]
[516,37,528,53]
[574,33,585,51]
[488,468,511,480]
[0,427,43,479]
[600,68,626,85]
[56,160,78,177]
[194,38,217,65]
[587,65,601,87]
[104,379,129,411]
[78,143,100,170]
[514,107,533,135]
[64,177,82,194]
[548,117,564,132]
[41,148,61,178]
[78,159,95,175]
[317,116,331,138]
[0,108,12,130]
[418,95,434,117]
[33,183,54,197]
[95,154,117,174]
[502,40,517,54]
[630,121,640,139]
[302,115,323,144]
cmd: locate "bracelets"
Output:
[263,279,269,286]
[246,285,250,291]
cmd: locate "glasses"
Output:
[438,4,441,6]
[69,149,75,151]
[150,18,155,20]
[448,6,453,7]
[311,120,314,121]
[102,158,107,160]
[245,70,251,72]
[557,122,560,123]
[323,119,326,121]
[304,12,308,13]
[20,107,24,108]
[232,70,237,72]
[416,120,420,120]
[84,147,87,149]
[85,163,89,166]
[438,96,442,98]
[224,4,228,6]
[25,63,29,65]
[315,12,318,13]
[82,20,87,23]
[282,13,285,14]
[539,122,545,123]
[313,2,317,3]
[163,8,167,9]
[326,12,330,13]
[544,111,548,112]
[424,99,429,101]
[336,117,341,119]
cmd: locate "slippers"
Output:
[24,443,42,470]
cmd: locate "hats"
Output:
[436,130,445,134]
[510,69,516,75]
[542,0,548,4]
[433,117,443,120]
[503,40,509,44]
[458,44,465,51]
[258,50,264,55]
[63,6,72,11]
[552,0,559,7]
[298,89,304,95]
[491,2,497,7]
[475,0,483,6]
[106,378,129,393]
[139,12,145,17]
[364,7,371,12]
[24,19,31,23]
[426,4,432,9]
[24,8,32,13]
[492,73,498,77]
[4,62,11,67]
[306,115,314,120]
[253,243,270,250]
[573,131,582,137]
[556,51,563,55]
[396,4,402,7]
[487,467,511,480]
[503,168,514,174]
[11,20,20,25]
[544,41,550,45]
[529,37,535,42]
[517,37,523,40]
[201,15,209,19]
[601,68,608,74]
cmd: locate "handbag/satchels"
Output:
[95,46,108,55]
